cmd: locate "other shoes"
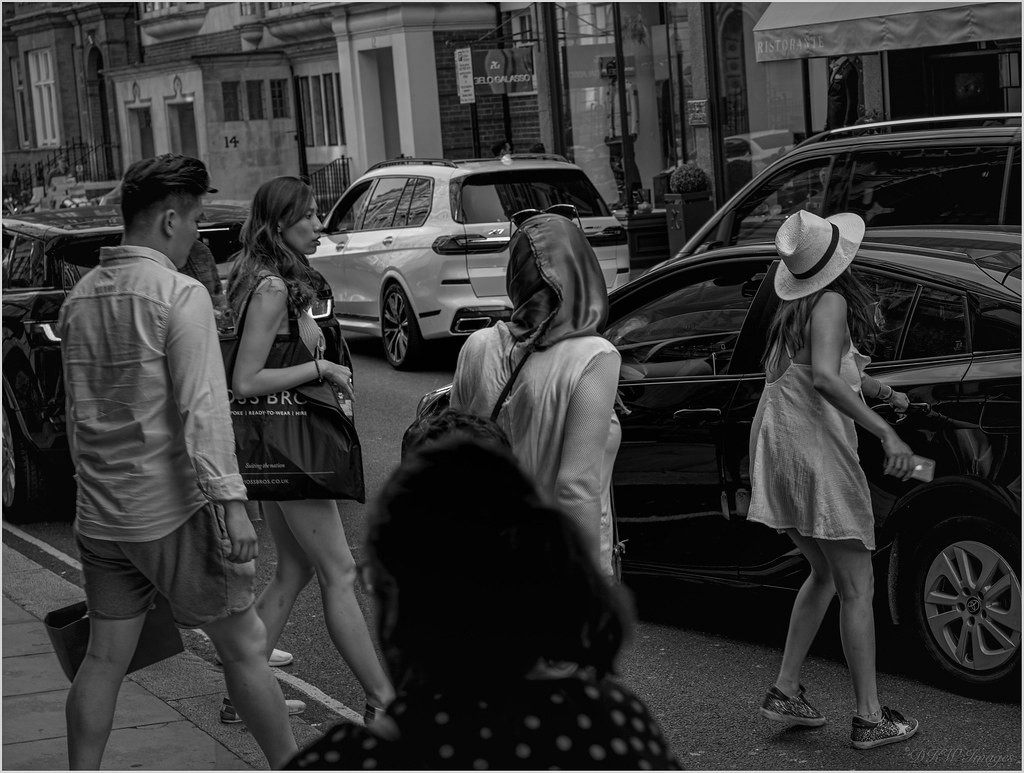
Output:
[219,694,307,723]
[214,648,293,666]
[364,703,387,725]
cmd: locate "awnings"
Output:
[752,2,1021,62]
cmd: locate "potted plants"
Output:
[663,164,715,258]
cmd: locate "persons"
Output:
[48,155,75,190]
[741,210,918,751]
[448,217,624,592]
[177,176,400,729]
[63,154,300,770]
[277,436,688,772]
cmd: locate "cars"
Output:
[416,238,1022,707]
[721,128,798,164]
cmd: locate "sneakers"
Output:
[760,683,826,726]
[850,705,919,750]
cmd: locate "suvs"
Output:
[306,150,633,374]
[676,108,1021,245]
[2,173,254,530]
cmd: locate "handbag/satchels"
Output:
[218,274,366,505]
[43,587,185,684]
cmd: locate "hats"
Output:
[121,152,220,194]
[774,209,866,302]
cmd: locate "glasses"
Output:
[509,203,582,237]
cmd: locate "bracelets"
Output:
[873,379,881,398]
[881,385,894,402]
[311,358,322,381]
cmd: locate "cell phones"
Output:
[909,454,936,483]
